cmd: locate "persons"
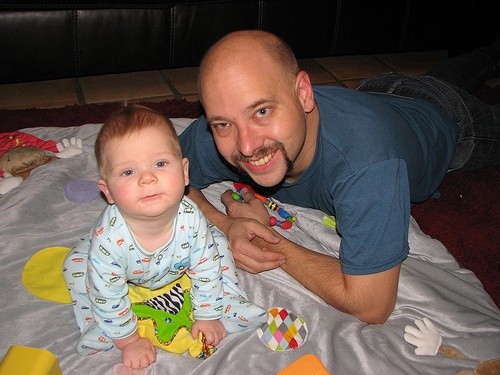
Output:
[65,105,269,367]
[176,30,500,323]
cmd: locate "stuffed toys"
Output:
[0,136,85,192]
[404,318,469,362]
